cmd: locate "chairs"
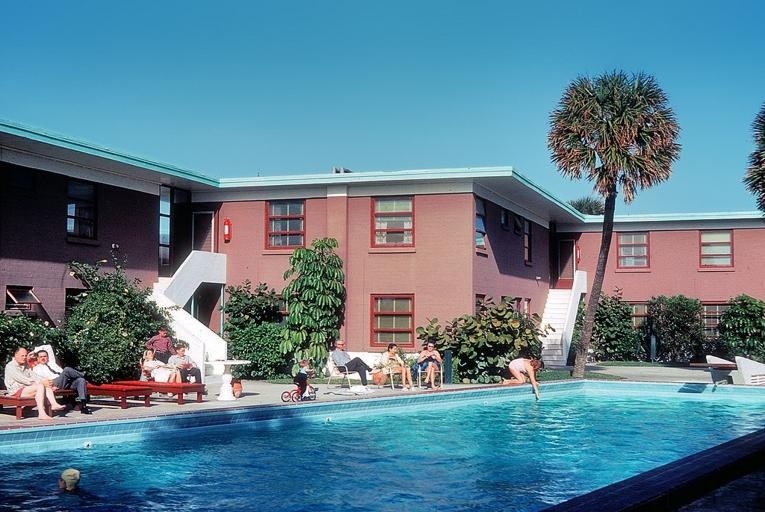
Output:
[378,355,400,390]
[139,358,165,397]
[28,355,80,411]
[328,357,366,395]
[415,355,443,389]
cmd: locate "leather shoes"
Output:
[82,406,93,414]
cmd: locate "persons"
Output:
[417,340,443,390]
[142,350,182,384]
[168,344,209,395]
[294,360,314,396]
[144,326,178,364]
[503,357,542,400]
[379,343,417,392]
[332,340,373,386]
[58,467,85,494]
[4,347,67,422]
[26,343,102,415]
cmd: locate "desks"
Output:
[202,360,251,400]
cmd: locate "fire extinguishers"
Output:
[224,216,232,240]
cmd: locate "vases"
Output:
[231,378,243,398]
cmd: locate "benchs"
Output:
[85,382,153,409]
[1,393,53,420]
[112,380,205,405]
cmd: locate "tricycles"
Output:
[280,374,319,403]
